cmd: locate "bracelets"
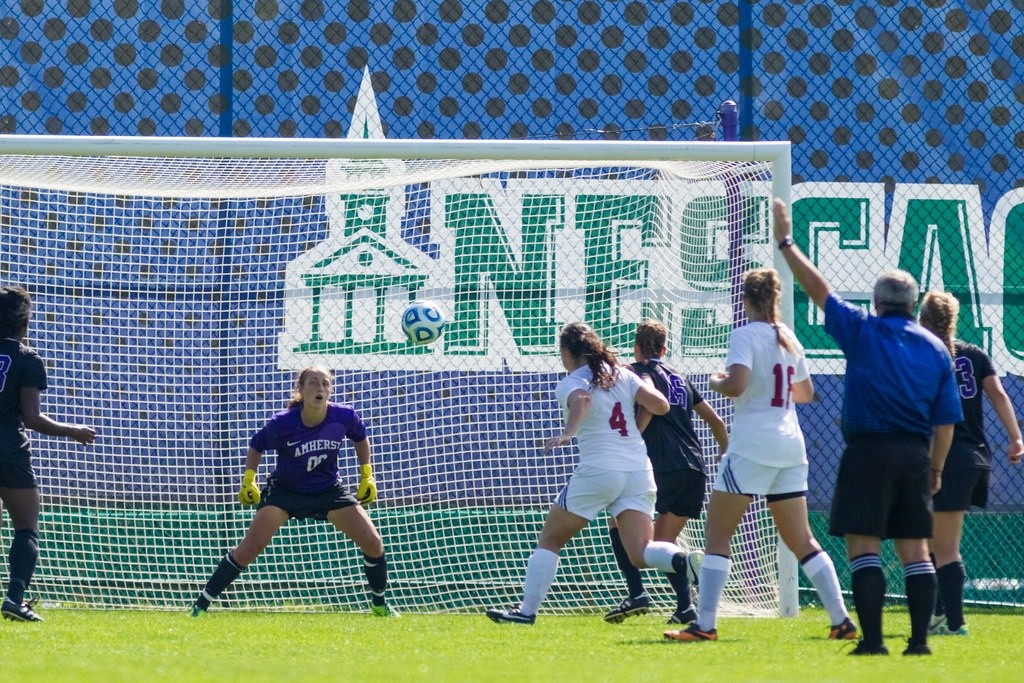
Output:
[779,239,795,250]
[932,469,943,475]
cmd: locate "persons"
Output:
[663,268,859,641]
[916,289,1024,635]
[0,286,96,623]
[485,323,704,627]
[603,319,730,626]
[773,196,964,655]
[189,366,401,618]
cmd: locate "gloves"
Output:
[237,469,261,505]
[356,464,377,508]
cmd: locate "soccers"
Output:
[401,299,446,346]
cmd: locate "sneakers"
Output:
[191,605,205,619]
[663,620,717,642]
[371,600,401,618]
[681,549,705,596]
[927,614,948,635]
[1,593,45,622]
[824,617,859,640]
[486,605,536,627]
[666,604,700,624]
[948,624,968,635]
[603,591,650,625]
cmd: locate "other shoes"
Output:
[902,638,931,655]
[848,635,888,654]
